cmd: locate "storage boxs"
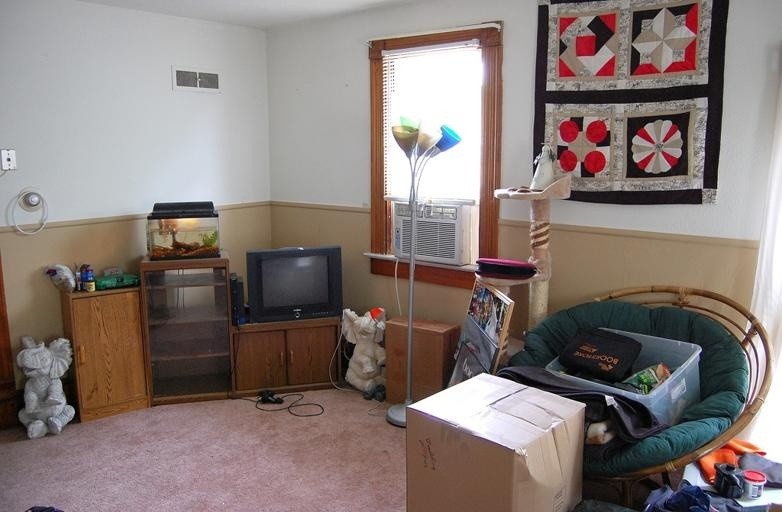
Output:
[544,324,702,432]
[403,373,587,511]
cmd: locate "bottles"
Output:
[76,272,82,291]
[87,269,96,292]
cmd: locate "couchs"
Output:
[499,284,778,512]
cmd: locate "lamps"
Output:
[386,116,463,429]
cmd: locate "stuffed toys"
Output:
[342,306,385,395]
[18,335,75,439]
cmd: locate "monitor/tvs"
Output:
[246,245,342,323]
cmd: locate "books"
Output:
[448,278,514,390]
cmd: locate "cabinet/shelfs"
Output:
[59,284,150,423]
[230,316,343,399]
[385,317,461,408]
[140,251,234,408]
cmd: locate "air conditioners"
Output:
[392,196,480,266]
